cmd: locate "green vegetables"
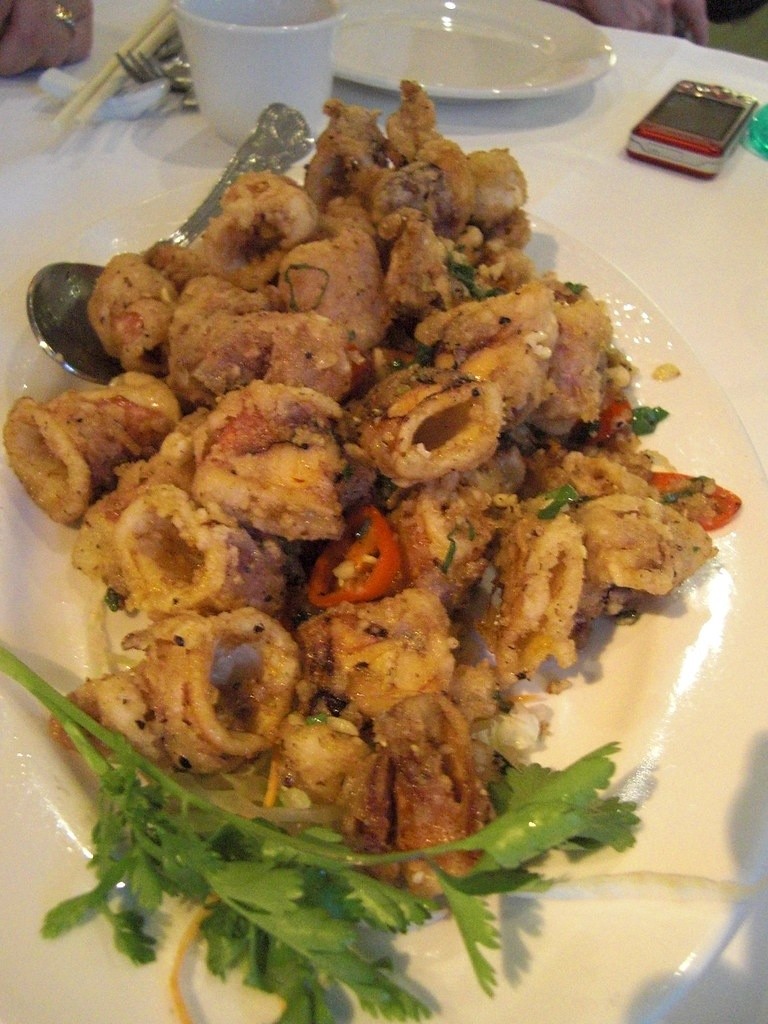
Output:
[1,644,644,1023]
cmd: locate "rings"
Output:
[55,3,73,20]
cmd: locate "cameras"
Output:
[627,80,758,181]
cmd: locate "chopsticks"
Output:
[57,0,179,129]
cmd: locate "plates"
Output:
[1,179,762,1024]
[334,1,615,100]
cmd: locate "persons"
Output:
[550,0,711,47]
[0,0,93,76]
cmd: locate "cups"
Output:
[171,1,347,150]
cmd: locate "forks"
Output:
[115,33,193,92]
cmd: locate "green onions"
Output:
[283,251,668,573]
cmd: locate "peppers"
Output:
[305,507,397,605]
[599,401,742,529]
